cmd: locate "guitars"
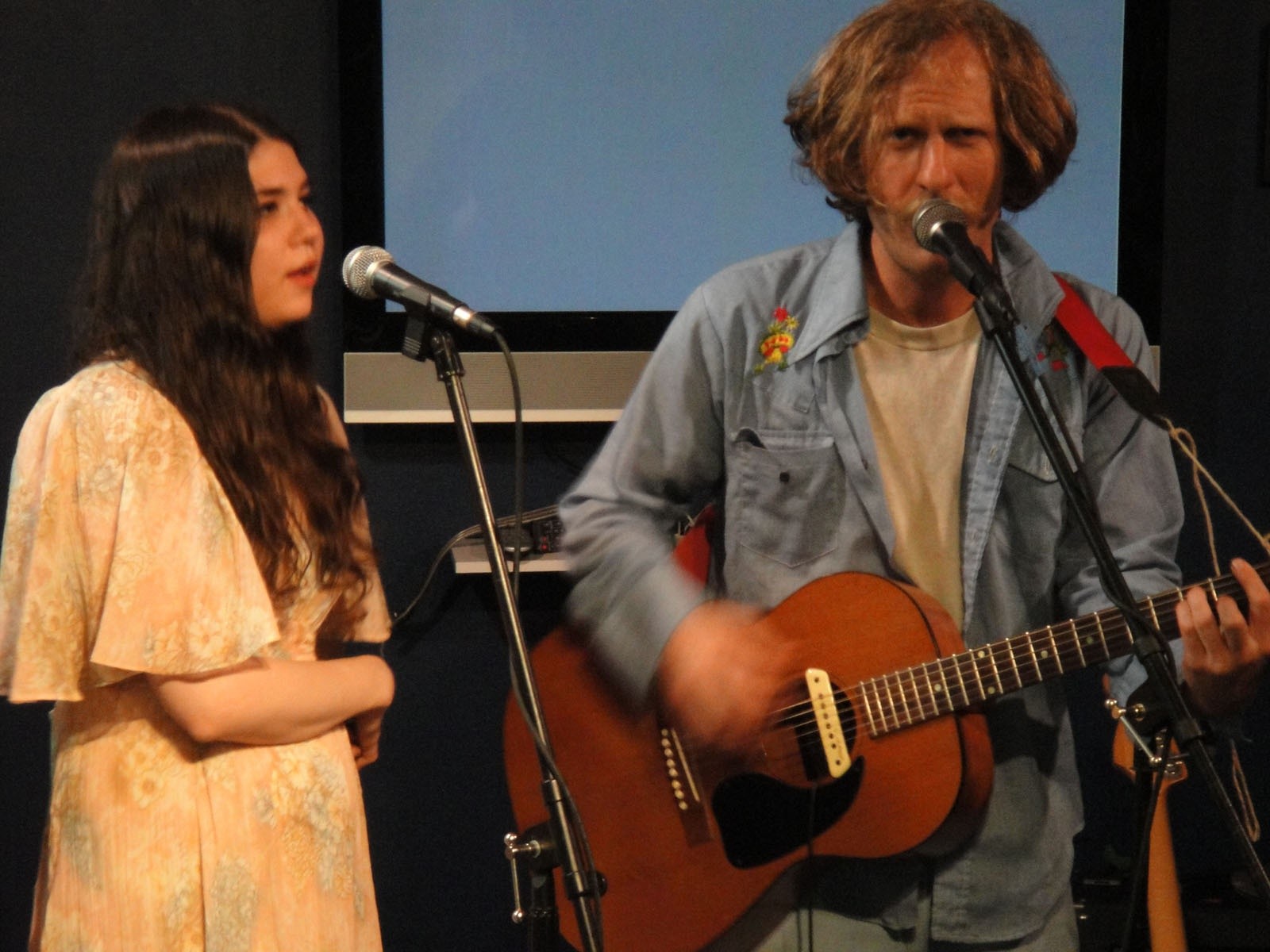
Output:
[503,562,1269,952]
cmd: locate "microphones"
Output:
[911,199,1023,325]
[342,245,498,338]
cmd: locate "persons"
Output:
[1,98,393,952]
[541,0,1270,951]
